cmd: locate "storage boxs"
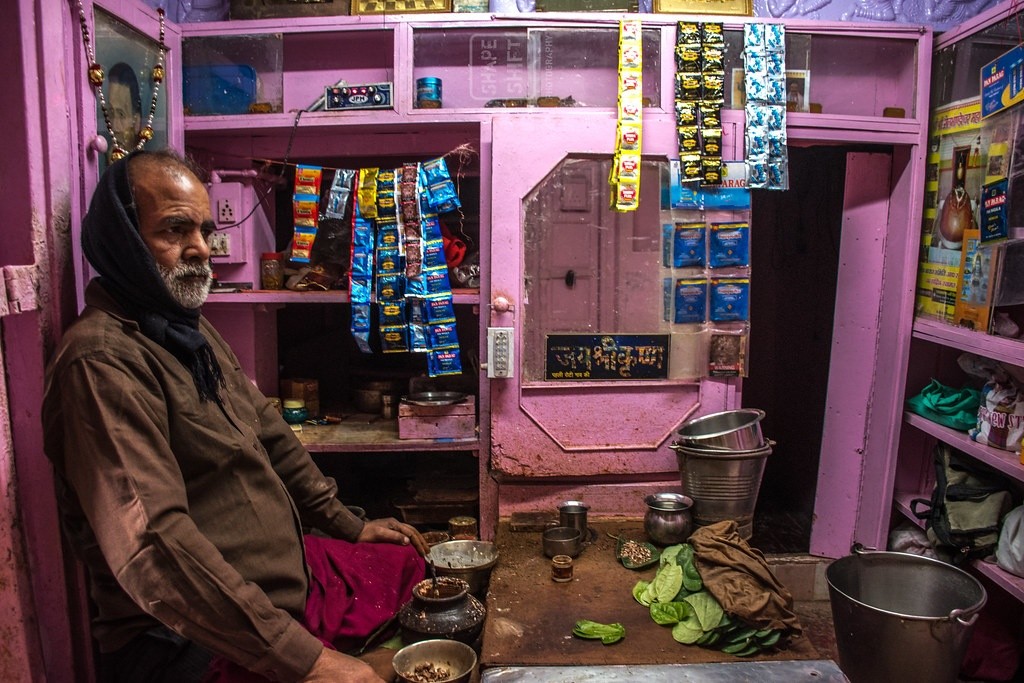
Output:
[397,396,477,440]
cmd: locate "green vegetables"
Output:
[574,542,780,655]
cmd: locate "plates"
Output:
[400,392,464,406]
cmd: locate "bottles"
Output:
[399,577,487,648]
[283,398,308,424]
[380,394,394,419]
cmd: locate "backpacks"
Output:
[910,441,1016,565]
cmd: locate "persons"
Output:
[42,151,431,683]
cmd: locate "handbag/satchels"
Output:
[907,376,982,430]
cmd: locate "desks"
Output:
[480,521,848,683]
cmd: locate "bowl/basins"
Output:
[424,540,499,594]
[350,382,394,412]
[557,500,591,540]
[542,527,581,558]
[643,493,693,546]
[676,408,766,451]
[392,639,477,683]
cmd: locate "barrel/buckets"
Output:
[824,540,988,683]
[667,437,773,540]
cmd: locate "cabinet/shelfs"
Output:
[0,0,1024,683]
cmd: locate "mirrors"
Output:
[522,153,702,381]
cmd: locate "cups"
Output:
[416,77,443,109]
[550,554,574,583]
